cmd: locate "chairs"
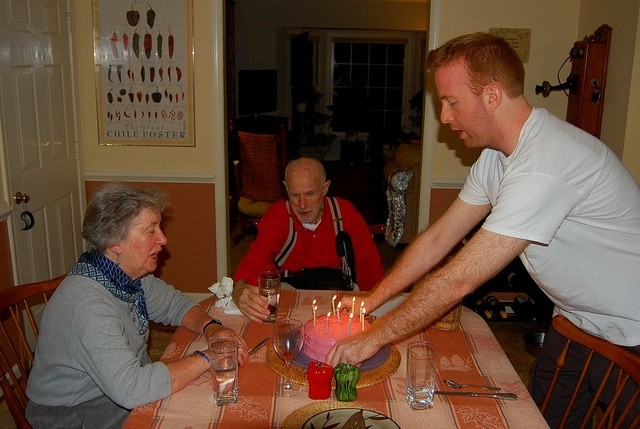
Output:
[537,311,640,428]
[229,119,289,244]
[1,276,75,429]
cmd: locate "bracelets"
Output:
[202,319,223,334]
[194,349,210,364]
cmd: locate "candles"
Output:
[312,300,317,310]
[325,312,331,335]
[336,302,343,322]
[347,312,354,335]
[331,295,337,315]
[351,296,356,314]
[312,305,318,326]
[361,308,366,331]
[359,300,364,321]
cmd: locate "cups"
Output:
[208,337,240,406]
[258,271,281,323]
[405,341,438,411]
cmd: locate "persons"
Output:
[324,31,639,428]
[231,156,386,325]
[24,182,250,429]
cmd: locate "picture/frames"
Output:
[89,0,198,148]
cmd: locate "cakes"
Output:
[302,312,372,362]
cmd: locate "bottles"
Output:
[504,271,528,290]
[486,295,507,318]
[514,295,537,321]
[474,297,493,319]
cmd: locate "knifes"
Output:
[249,337,270,357]
[434,390,518,401]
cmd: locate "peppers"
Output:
[307,361,333,400]
[334,363,360,401]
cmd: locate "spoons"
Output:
[445,379,501,391]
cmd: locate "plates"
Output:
[300,408,402,429]
[282,326,390,372]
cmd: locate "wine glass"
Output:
[273,318,305,397]
